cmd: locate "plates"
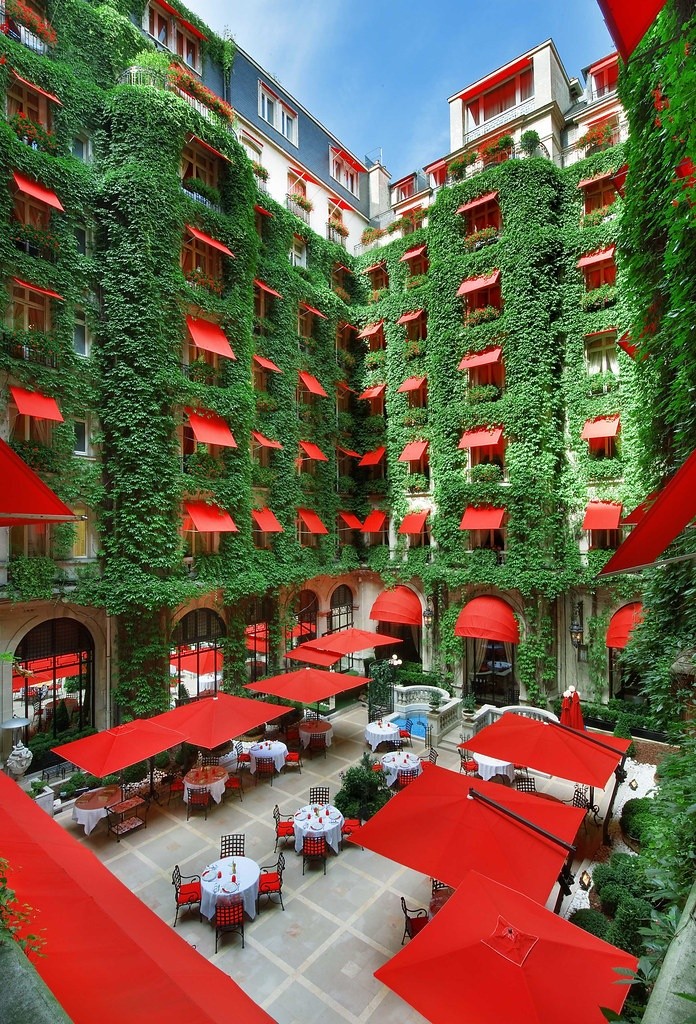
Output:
[383,757,391,762]
[224,882,238,892]
[202,873,215,880]
[295,805,340,830]
[400,763,410,768]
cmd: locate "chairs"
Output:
[168,716,590,953]
[14,677,79,726]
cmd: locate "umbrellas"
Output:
[347,690,641,1023]
[51,627,403,778]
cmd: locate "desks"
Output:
[183,765,230,803]
[200,856,260,919]
[380,750,423,787]
[526,791,565,805]
[71,785,124,837]
[44,697,78,719]
[197,673,222,691]
[429,889,454,916]
[299,720,333,749]
[365,720,402,752]
[249,740,289,775]
[472,751,515,783]
[294,804,345,854]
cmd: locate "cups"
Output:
[232,875,236,882]
[326,810,330,815]
[308,813,311,819]
[218,871,221,878]
[319,817,322,823]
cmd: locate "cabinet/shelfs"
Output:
[105,793,149,843]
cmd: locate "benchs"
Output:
[42,764,67,785]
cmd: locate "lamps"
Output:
[422,607,433,629]
[568,620,584,648]
[579,871,592,890]
[629,779,638,789]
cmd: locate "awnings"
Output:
[0,0,696,649]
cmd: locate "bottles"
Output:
[233,863,236,874]
